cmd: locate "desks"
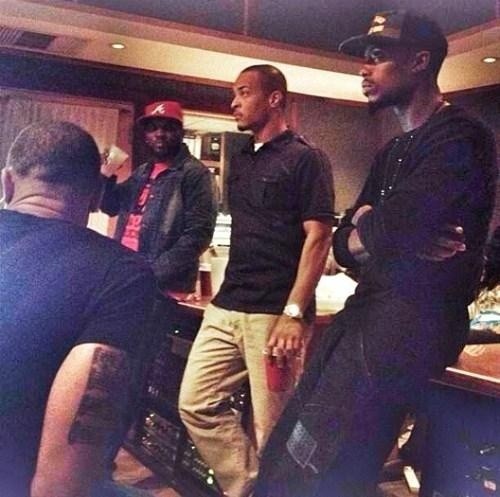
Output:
[103,240,500,497]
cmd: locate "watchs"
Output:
[281,303,304,321]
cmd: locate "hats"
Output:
[338,7,448,62]
[137,99,184,127]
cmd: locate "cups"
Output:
[264,346,292,392]
[100,144,129,178]
[198,263,212,298]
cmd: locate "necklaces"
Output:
[379,95,446,200]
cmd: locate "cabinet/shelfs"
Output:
[193,131,255,214]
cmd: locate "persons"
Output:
[0,119,155,496]
[100,100,220,483]
[252,8,496,496]
[176,62,337,497]
